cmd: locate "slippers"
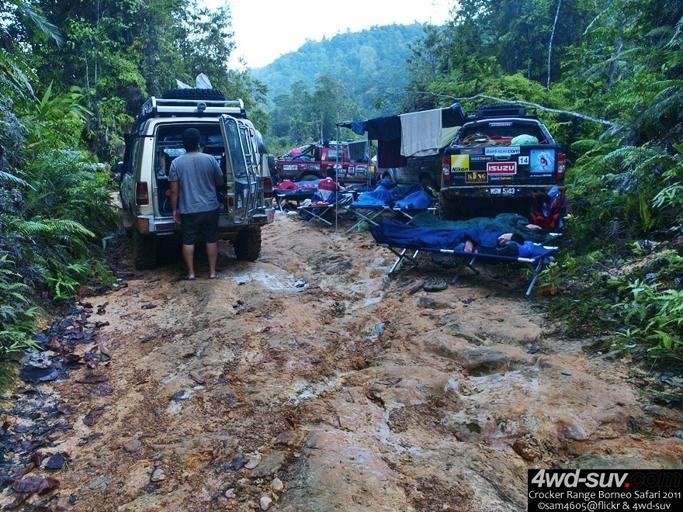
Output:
[181,274,196,280]
[208,272,220,279]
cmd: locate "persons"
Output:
[525,222,548,237]
[463,232,524,253]
[167,128,226,283]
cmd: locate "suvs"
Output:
[110,99,274,268]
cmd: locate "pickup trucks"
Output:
[276,143,376,183]
[379,144,442,189]
[434,106,566,224]
